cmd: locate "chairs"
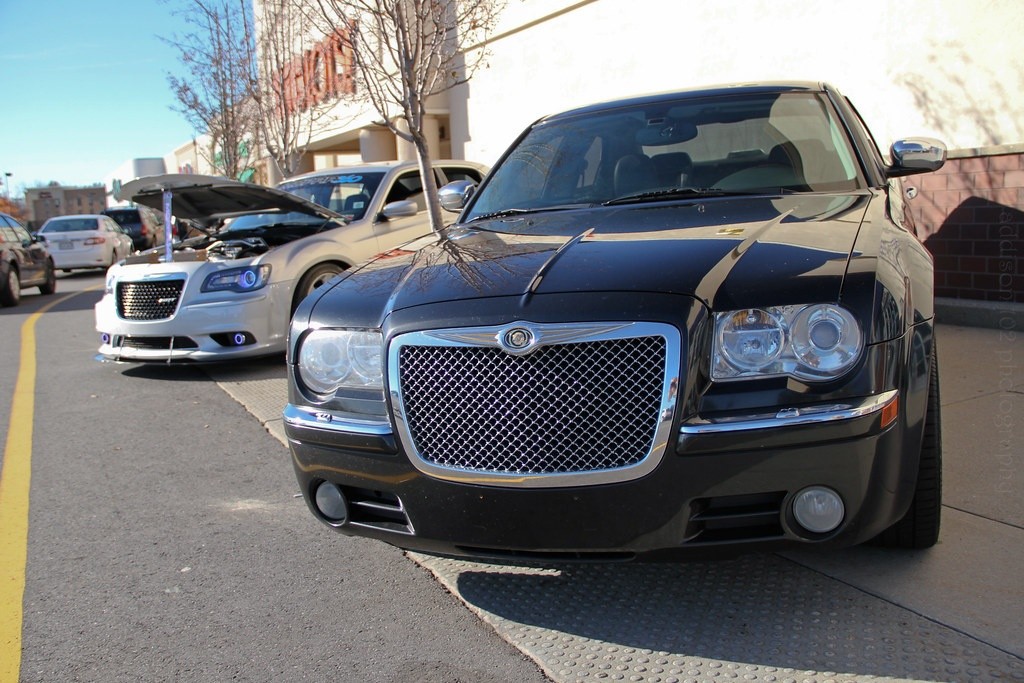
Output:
[613,138,847,195]
[344,195,371,220]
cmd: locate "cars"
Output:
[93,158,491,366]
[283,81,946,566]
[0,212,55,309]
[36,214,135,271]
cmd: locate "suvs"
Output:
[101,206,163,252]
